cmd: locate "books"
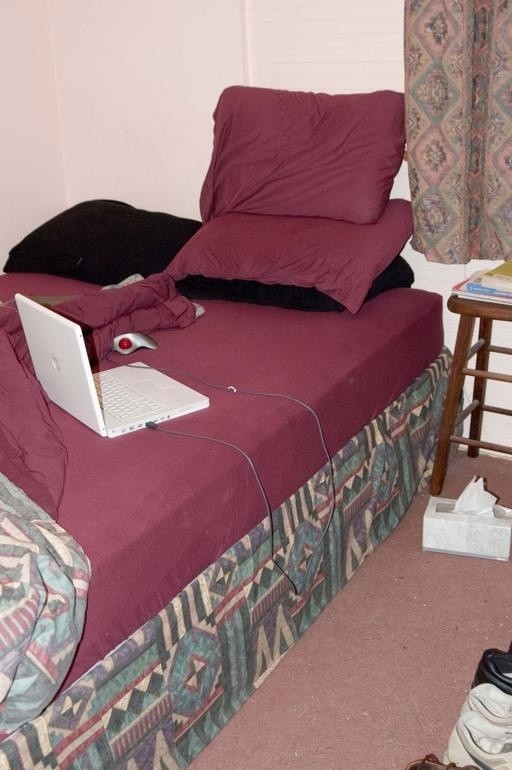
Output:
[452,258,512,305]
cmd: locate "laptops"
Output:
[15,293,210,439]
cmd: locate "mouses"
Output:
[110,331,158,355]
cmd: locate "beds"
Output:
[0,265,466,768]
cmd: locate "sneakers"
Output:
[446,649,511,770]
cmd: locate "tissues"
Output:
[421,475,512,561]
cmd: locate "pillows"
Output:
[200,81,410,229]
[166,196,415,317]
[6,200,415,310]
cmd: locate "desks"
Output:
[429,280,511,498]
